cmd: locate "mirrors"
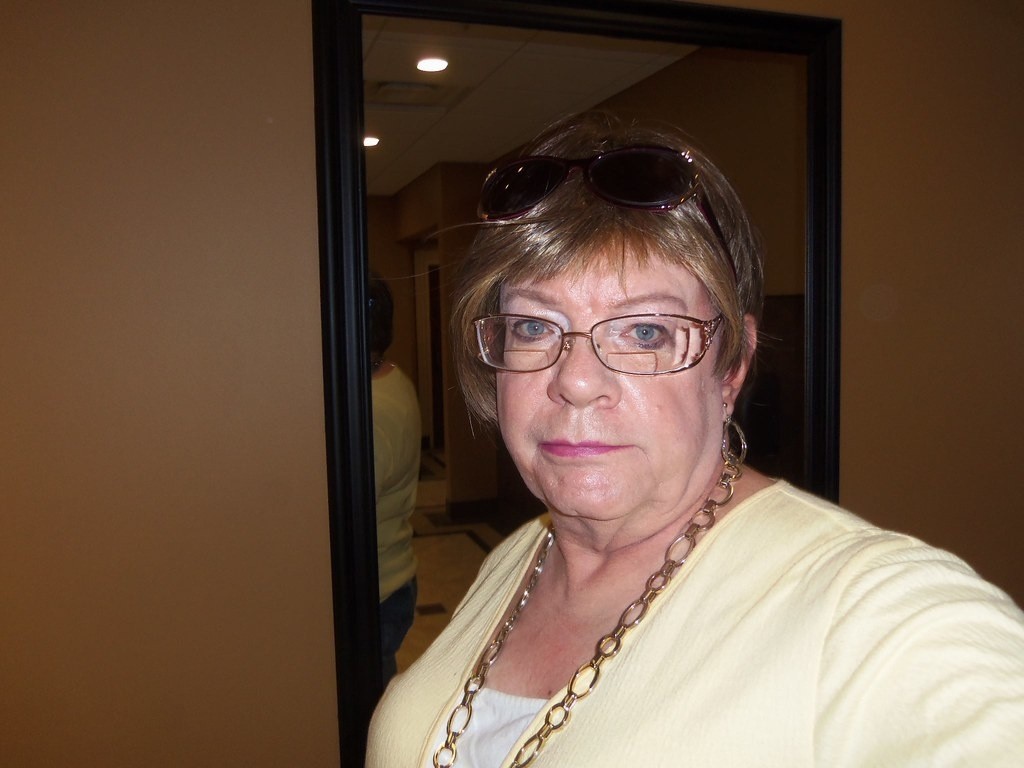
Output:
[311,0,841,768]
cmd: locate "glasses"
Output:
[477,143,738,289]
[471,312,723,375]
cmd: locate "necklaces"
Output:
[433,452,743,768]
[372,356,383,369]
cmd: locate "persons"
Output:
[366,261,423,688]
[360,121,1024,768]
